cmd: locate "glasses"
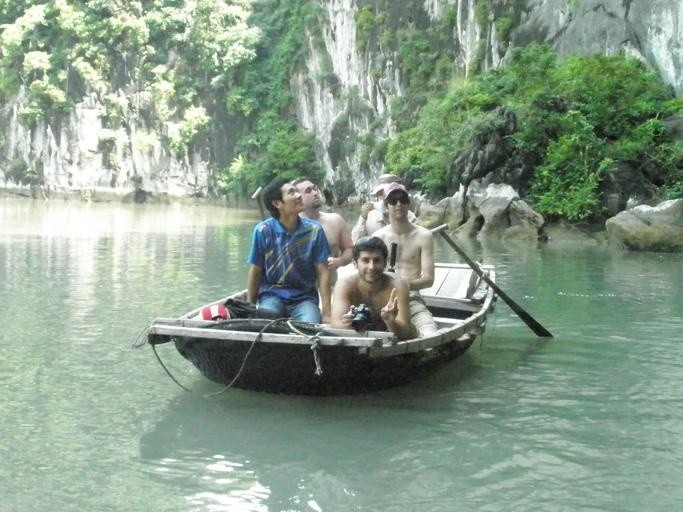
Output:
[385,197,409,206]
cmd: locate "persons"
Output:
[247,177,331,335]
[373,182,437,338]
[289,177,354,316]
[332,236,417,340]
[351,174,420,245]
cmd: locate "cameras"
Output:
[350,304,372,330]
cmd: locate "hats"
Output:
[368,172,404,196]
[383,180,409,200]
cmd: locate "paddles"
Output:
[440,231,553,338]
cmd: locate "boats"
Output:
[129,259,494,394]
